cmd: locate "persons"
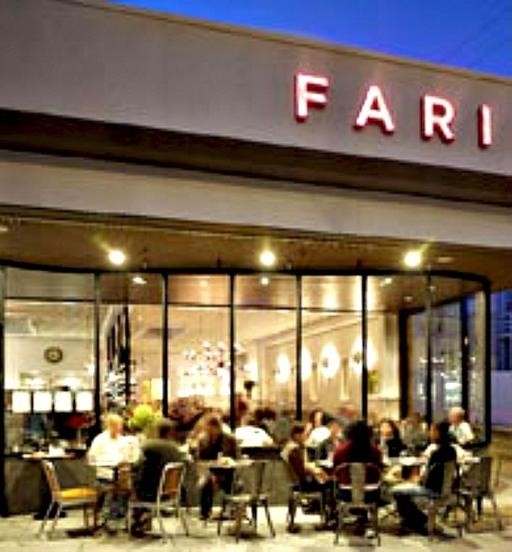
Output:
[36,395,476,539]
